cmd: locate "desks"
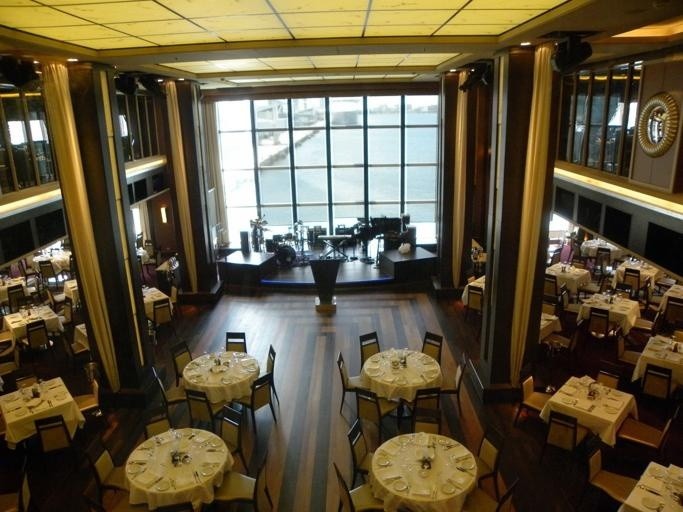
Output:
[215,250,277,281]
[380,247,440,279]
[317,234,353,261]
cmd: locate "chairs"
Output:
[145,417,171,440]
[225,332,248,352]
[616,404,680,465]
[84,436,129,505]
[464,477,519,511]
[232,372,277,435]
[536,409,590,479]
[219,416,250,473]
[167,341,193,386]
[637,361,672,419]
[216,449,276,511]
[333,462,384,511]
[644,276,664,313]
[468,231,681,366]
[184,390,221,434]
[0,235,182,374]
[348,419,373,489]
[578,449,638,511]
[261,345,281,407]
[473,426,506,502]
[543,318,587,366]
[441,352,467,418]
[513,374,552,431]
[360,332,382,369]
[421,331,443,367]
[408,386,442,430]
[150,365,186,417]
[354,387,402,435]
[337,352,368,414]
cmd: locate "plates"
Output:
[398,435,409,442]
[440,484,456,493]
[126,465,141,473]
[222,378,229,384]
[200,467,215,476]
[461,460,474,469]
[562,395,572,404]
[376,456,389,466]
[649,466,664,478]
[639,496,657,508]
[603,407,616,414]
[155,481,170,491]
[142,442,152,449]
[438,438,450,445]
[392,479,409,490]
